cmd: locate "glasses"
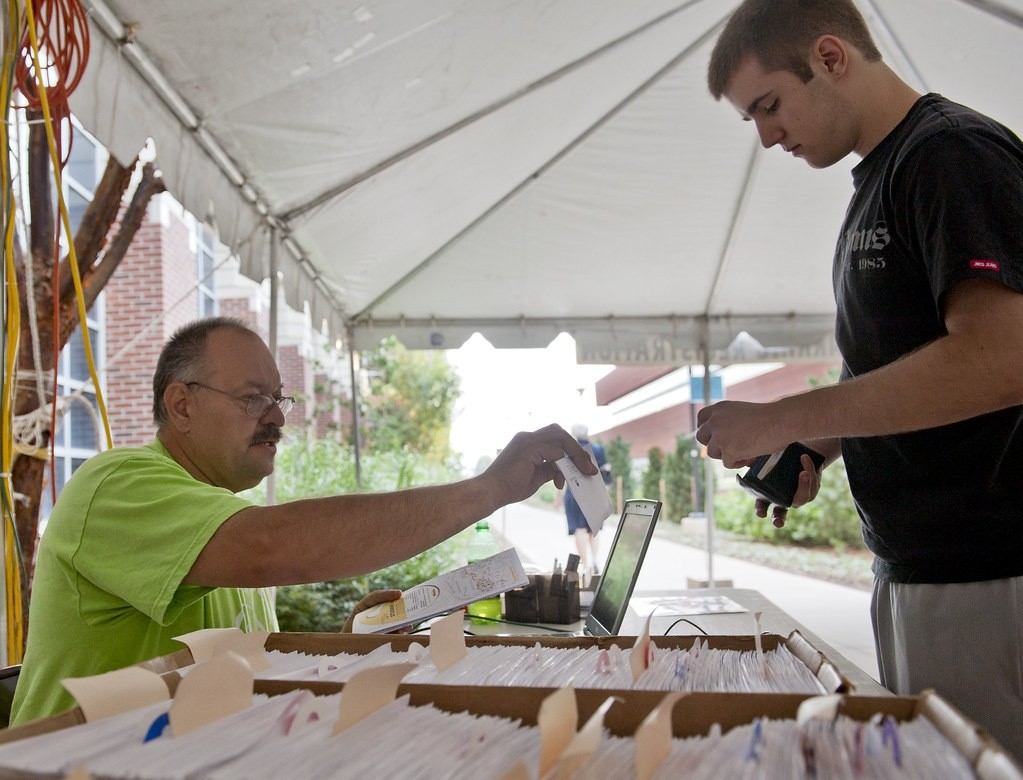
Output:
[183,380,296,419]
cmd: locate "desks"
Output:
[415,586,902,698]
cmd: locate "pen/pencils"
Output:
[548,558,558,595]
[554,563,562,595]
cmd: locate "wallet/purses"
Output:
[735,434,826,508]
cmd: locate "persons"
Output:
[695,0,1023,770]
[8,316,598,730]
[562,425,611,576]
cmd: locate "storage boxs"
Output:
[139,628,858,695]
[1,689,1023,780]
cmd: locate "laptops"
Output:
[490,496,664,642]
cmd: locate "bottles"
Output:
[467,521,502,624]
